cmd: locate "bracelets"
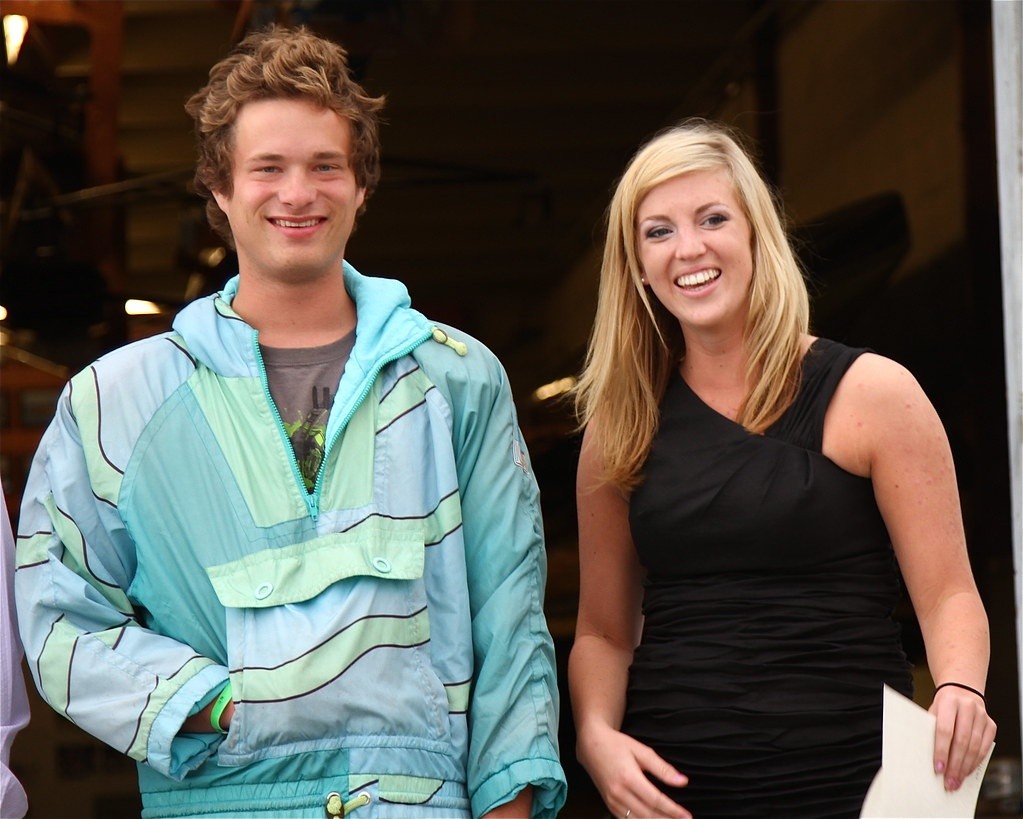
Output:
[931,683,988,708]
[211,683,232,735]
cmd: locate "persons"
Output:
[567,122,998,819]
[13,30,567,819]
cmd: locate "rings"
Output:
[625,809,630,819]
